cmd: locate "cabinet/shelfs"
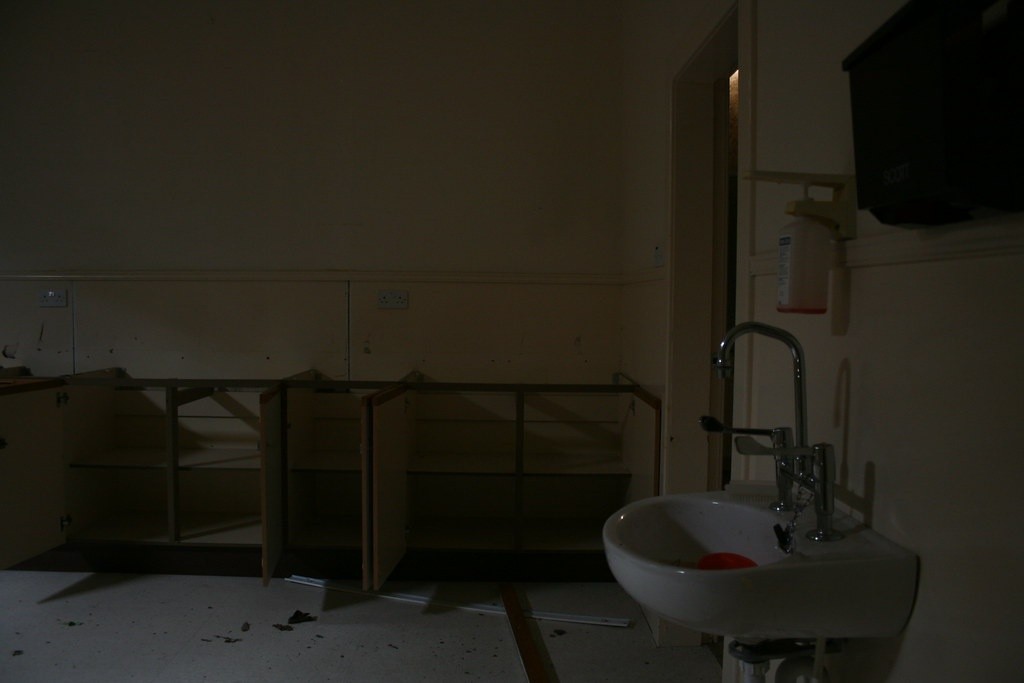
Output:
[0,365,662,593]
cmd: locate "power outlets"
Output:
[374,289,408,309]
[36,287,68,307]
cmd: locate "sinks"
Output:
[604,488,919,640]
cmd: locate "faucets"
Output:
[716,320,810,479]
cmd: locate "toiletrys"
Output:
[775,180,829,315]
